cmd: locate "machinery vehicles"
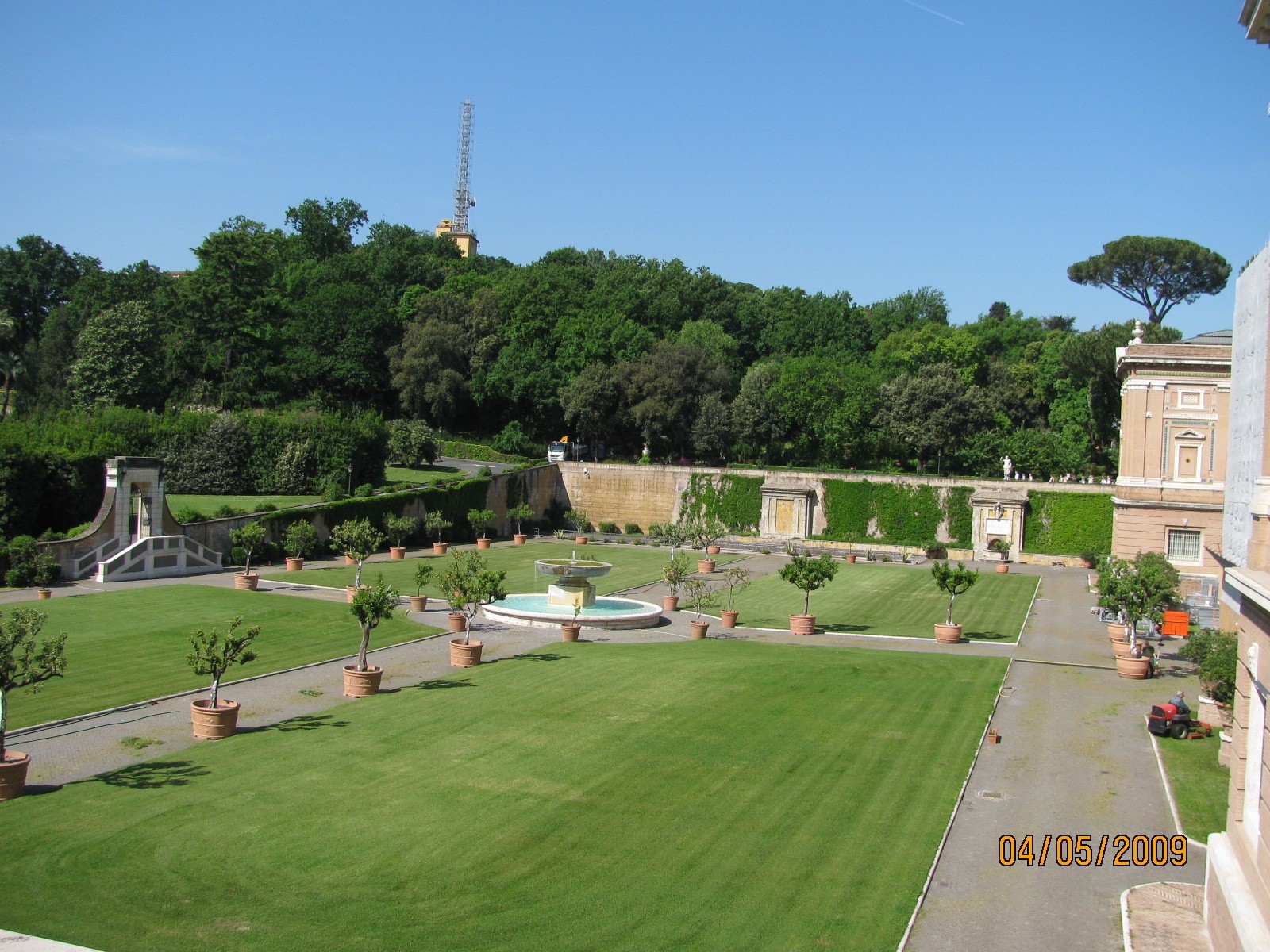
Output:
[1146,698,1212,741]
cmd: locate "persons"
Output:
[1117,608,1124,623]
[1142,640,1158,679]
[999,455,1012,481]
[1048,474,1068,483]
[1168,690,1192,714]
[1015,471,1033,481]
[1079,475,1111,484]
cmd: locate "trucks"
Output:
[547,436,588,462]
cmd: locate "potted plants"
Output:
[1179,626,1237,767]
[0,594,68,802]
[189,505,1179,741]
[24,542,58,599]
